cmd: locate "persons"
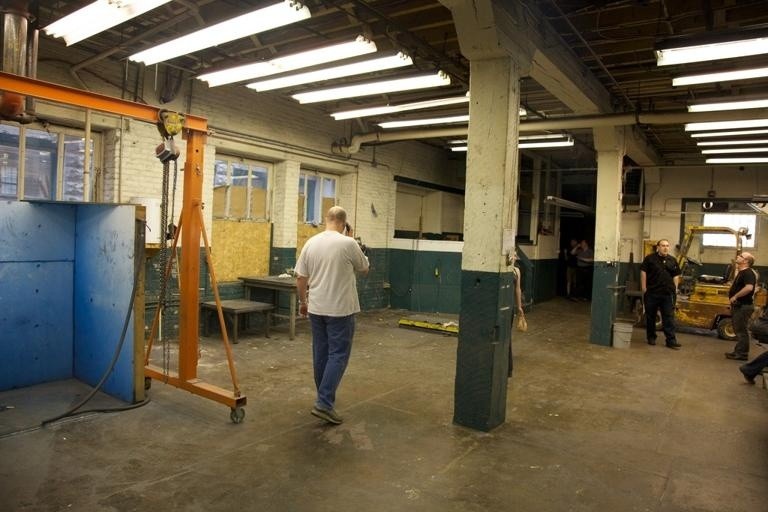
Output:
[508,251,527,378]
[295,206,370,424]
[564,238,579,300]
[740,350,768,384]
[725,252,757,361]
[570,238,594,300]
[640,239,682,348]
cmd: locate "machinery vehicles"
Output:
[625,226,767,340]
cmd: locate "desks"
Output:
[237,274,309,340]
[201,298,274,346]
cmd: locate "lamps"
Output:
[653,23,768,165]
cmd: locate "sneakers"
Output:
[740,367,756,384]
[725,352,749,361]
[666,343,681,347]
[648,338,656,345]
[311,403,343,424]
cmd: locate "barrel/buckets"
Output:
[612,323,633,349]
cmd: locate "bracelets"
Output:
[298,298,308,304]
